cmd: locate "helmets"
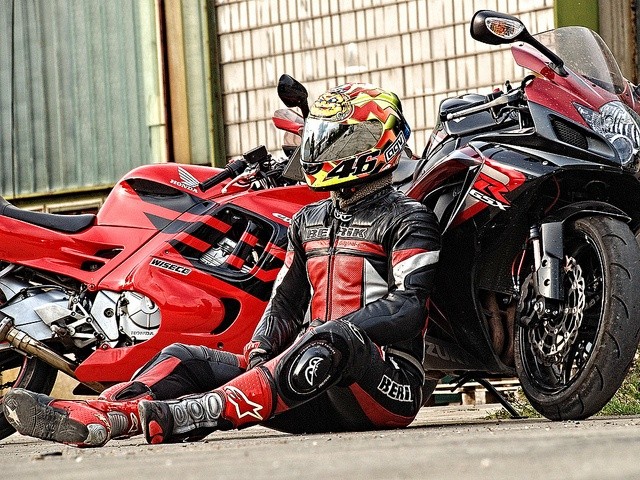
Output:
[300,83,411,192]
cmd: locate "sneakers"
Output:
[138,391,233,444]
[3,388,112,448]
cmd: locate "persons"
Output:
[2,82,443,448]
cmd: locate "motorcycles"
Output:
[0,74,310,441]
[226,8,640,422]
